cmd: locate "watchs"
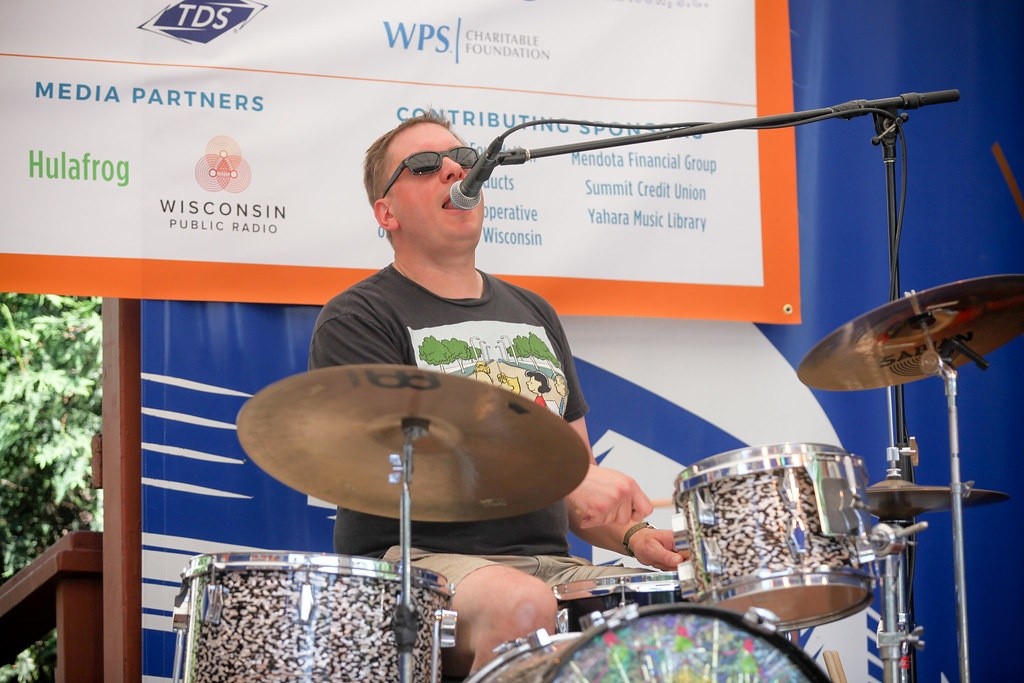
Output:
[622,522,659,558]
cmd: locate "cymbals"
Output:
[795,273,1024,392]
[855,478,1012,522]
[235,362,590,524]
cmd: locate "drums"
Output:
[550,571,684,635]
[671,442,876,636]
[169,550,458,683]
[461,602,835,683]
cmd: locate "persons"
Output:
[308,111,690,683]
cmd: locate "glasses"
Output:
[381,147,480,200]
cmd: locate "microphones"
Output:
[450,136,503,210]
